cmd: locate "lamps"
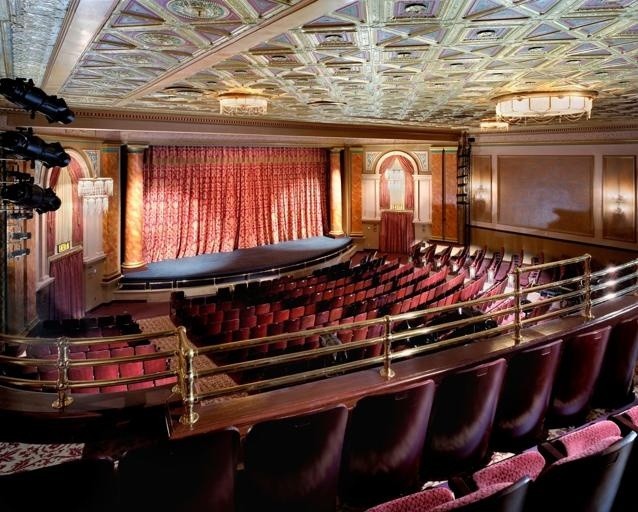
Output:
[478,89,600,133]
[76,128,114,204]
[218,92,273,116]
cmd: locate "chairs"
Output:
[2,240,634,509]
